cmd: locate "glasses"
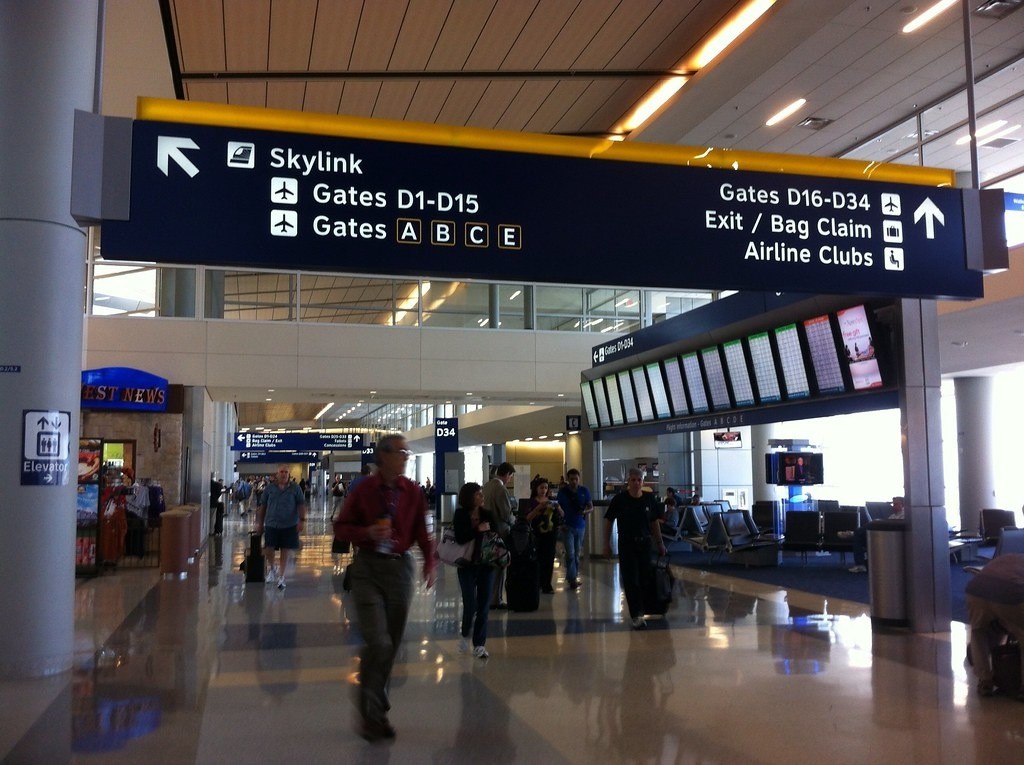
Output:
[387,449,413,457]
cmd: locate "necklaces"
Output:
[472,514,479,521]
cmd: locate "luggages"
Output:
[635,551,675,619]
[504,551,540,614]
[241,530,266,585]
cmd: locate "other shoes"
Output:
[264,565,277,583]
[543,582,554,595]
[490,602,507,610]
[359,692,395,742]
[977,676,993,696]
[631,616,647,629]
[570,580,582,590]
[838,530,855,541]
[473,643,490,658]
[850,564,867,574]
[275,574,286,590]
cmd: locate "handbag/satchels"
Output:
[508,516,531,558]
[472,530,511,572]
[432,527,474,568]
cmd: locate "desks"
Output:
[958,538,983,564]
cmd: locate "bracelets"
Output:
[299,517,305,521]
[582,510,585,514]
[531,510,537,516]
[559,510,563,514]
[659,539,664,544]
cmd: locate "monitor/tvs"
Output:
[766,453,824,486]
[713,432,742,448]
[580,303,887,428]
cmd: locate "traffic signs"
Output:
[233,431,364,463]
[101,96,984,301]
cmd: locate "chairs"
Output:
[662,498,1024,579]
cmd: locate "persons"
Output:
[963,552,1024,697]
[837,496,904,572]
[299,478,305,495]
[347,465,371,493]
[292,478,297,484]
[661,486,703,528]
[305,479,310,502]
[229,475,276,516]
[795,457,809,485]
[330,473,346,521]
[333,434,435,745]
[519,478,564,594]
[557,468,667,629]
[120,467,145,568]
[454,463,517,657]
[417,477,430,502]
[210,471,223,536]
[256,464,307,589]
[530,474,564,500]
